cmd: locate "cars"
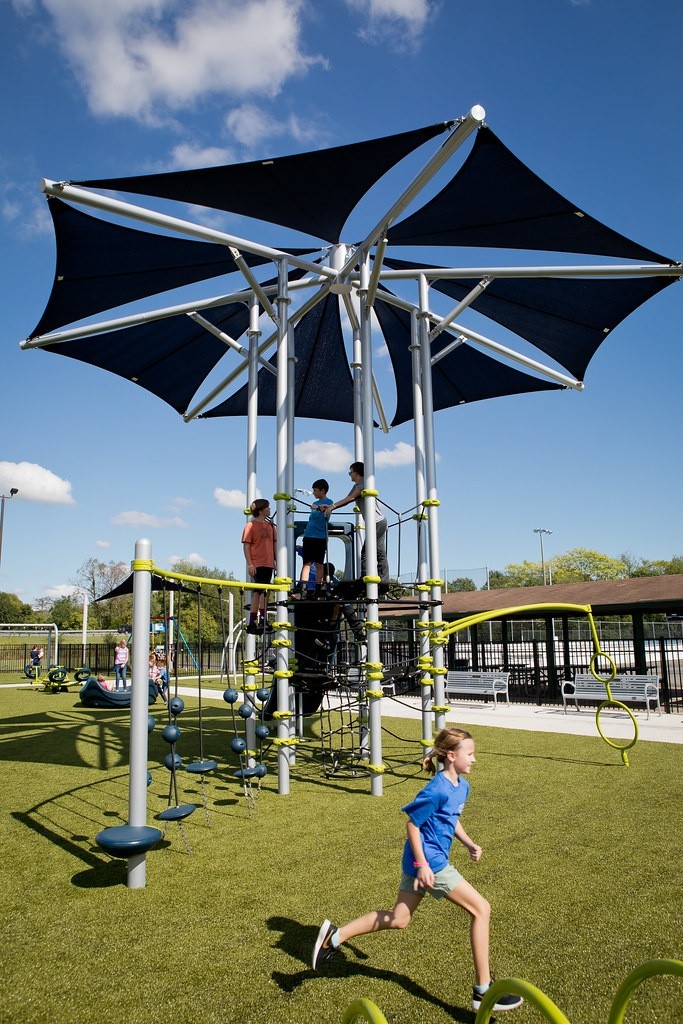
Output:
[118,624,132,635]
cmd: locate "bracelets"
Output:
[317,505,320,511]
[414,861,428,869]
[248,565,254,568]
[332,504,336,508]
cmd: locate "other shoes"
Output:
[248,619,264,631]
[258,619,273,631]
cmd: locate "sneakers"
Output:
[313,918,344,971]
[291,588,306,600]
[473,977,524,1010]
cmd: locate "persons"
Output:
[97,674,115,691]
[311,726,523,1011]
[289,463,391,649]
[114,639,129,692]
[149,651,170,705]
[241,499,276,634]
[29,645,44,677]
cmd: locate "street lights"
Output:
[533,527,553,586]
[0,488,19,564]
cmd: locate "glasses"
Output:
[348,472,352,476]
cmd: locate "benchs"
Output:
[348,668,396,696]
[444,671,510,710]
[561,674,662,720]
